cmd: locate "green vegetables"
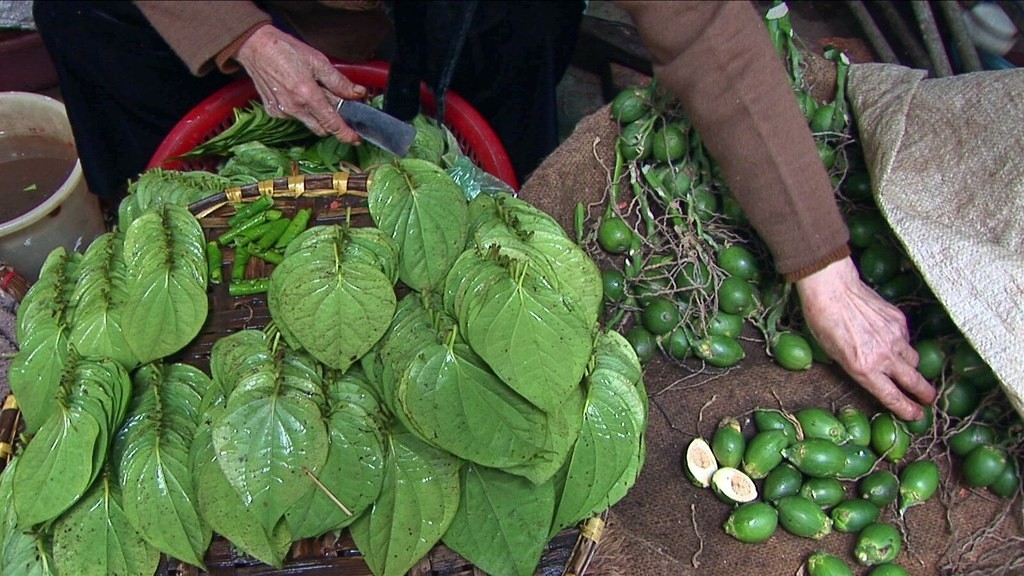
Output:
[0,88,651,576]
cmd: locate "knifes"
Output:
[321,87,417,158]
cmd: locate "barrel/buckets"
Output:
[0,90,104,284]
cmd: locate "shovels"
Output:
[326,89,417,159]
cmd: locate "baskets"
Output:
[0,173,611,576]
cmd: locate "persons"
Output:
[32,0,938,421]
